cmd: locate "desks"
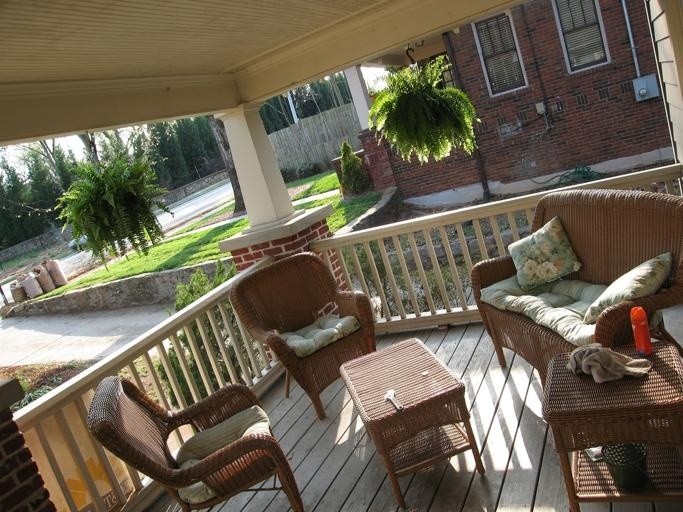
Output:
[340,338,485,510]
[542,341,683,512]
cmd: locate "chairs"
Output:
[87,376,304,512]
[230,251,376,419]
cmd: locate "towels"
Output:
[566,343,652,384]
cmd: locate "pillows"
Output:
[507,215,583,290]
[584,251,672,325]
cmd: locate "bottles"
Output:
[628,308,652,357]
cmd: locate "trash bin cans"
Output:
[601,442,648,490]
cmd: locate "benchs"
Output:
[471,190,683,389]
